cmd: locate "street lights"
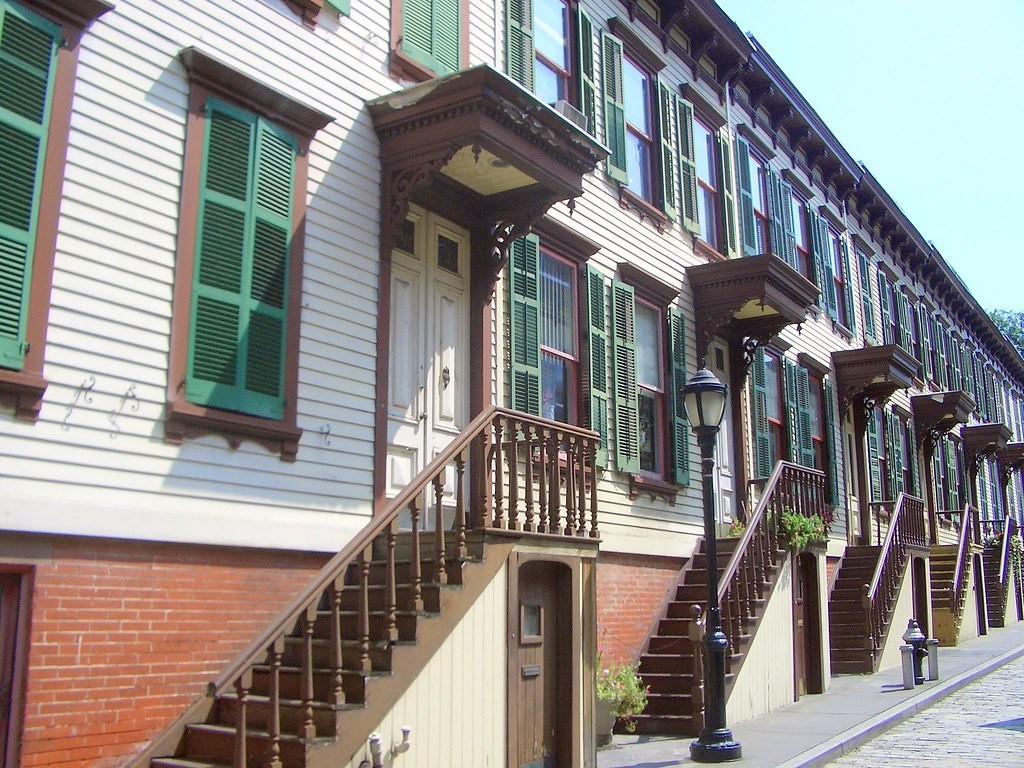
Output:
[681,357,743,763]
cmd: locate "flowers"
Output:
[594,644,651,733]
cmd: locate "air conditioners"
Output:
[549,99,588,133]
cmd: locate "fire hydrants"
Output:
[901,619,929,685]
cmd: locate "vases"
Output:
[594,700,615,748]
